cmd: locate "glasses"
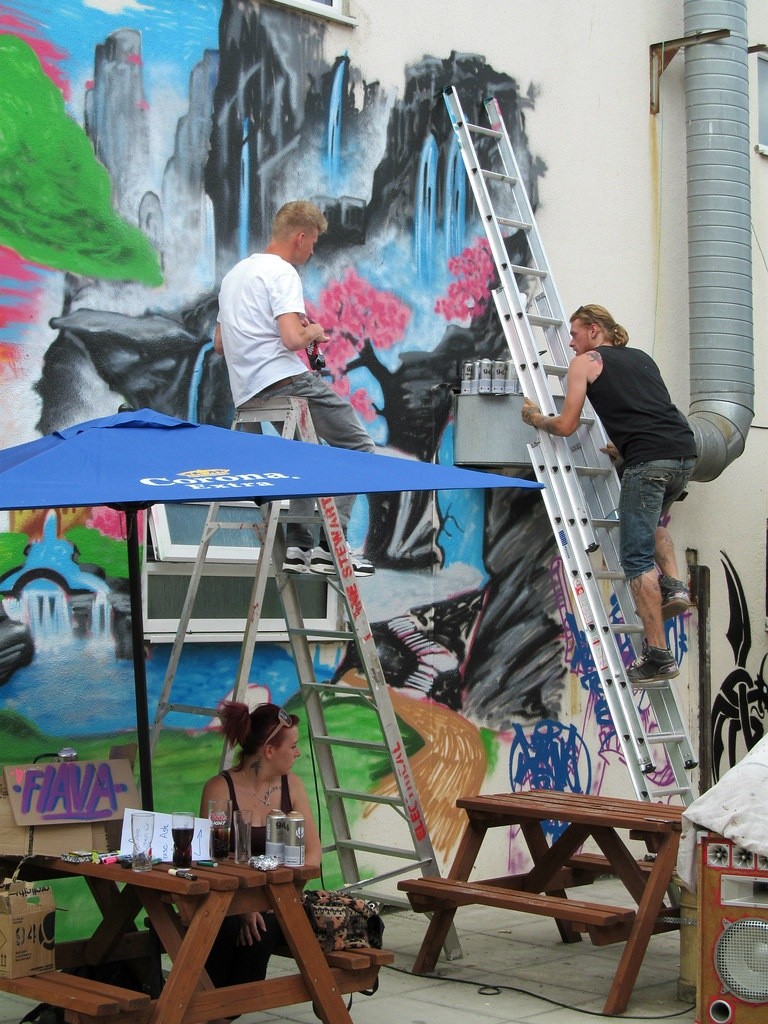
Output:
[264,708,292,751]
[575,306,603,329]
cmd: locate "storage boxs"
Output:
[0,742,143,857]
[0,877,56,980]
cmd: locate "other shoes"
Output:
[624,638,680,684]
[635,574,690,621]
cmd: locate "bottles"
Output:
[283,810,306,867]
[265,808,287,866]
[461,356,524,396]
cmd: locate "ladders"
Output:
[439,84,704,907]
[137,395,466,958]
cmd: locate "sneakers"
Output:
[281,547,314,575]
[310,546,376,577]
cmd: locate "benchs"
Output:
[0,912,393,1024]
[397,851,685,947]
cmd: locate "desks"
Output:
[10,838,356,1024]
[410,788,690,1017]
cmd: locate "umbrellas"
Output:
[0,405,547,814]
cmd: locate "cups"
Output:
[172,812,195,872]
[208,799,232,862]
[131,813,155,872]
[234,810,252,864]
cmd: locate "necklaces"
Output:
[241,769,272,806]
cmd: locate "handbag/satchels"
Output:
[303,889,385,1021]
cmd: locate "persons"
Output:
[521,304,699,683]
[213,202,377,576]
[197,700,322,1024]
